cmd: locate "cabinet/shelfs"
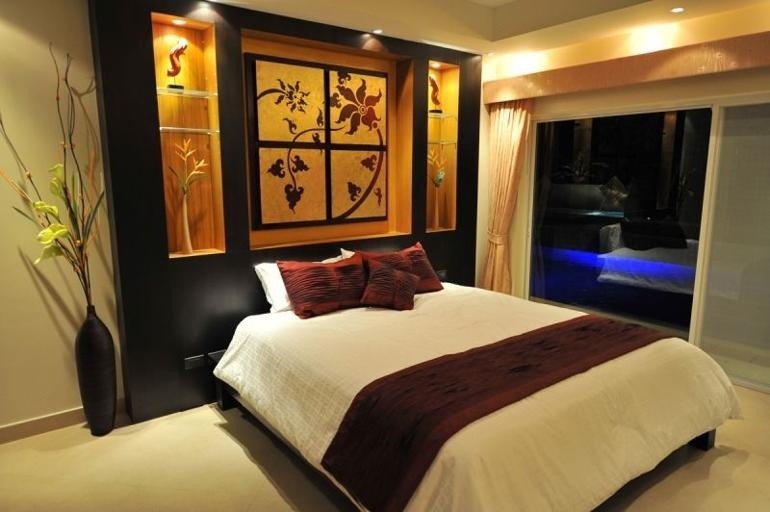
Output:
[151,12,227,260]
[426,61,458,234]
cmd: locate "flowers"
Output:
[0,39,108,309]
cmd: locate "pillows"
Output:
[358,242,443,293]
[340,246,353,263]
[277,255,369,320]
[359,258,420,310]
[254,257,345,312]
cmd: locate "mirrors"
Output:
[524,104,725,350]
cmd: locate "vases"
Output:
[76,305,117,438]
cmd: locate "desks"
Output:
[544,206,625,250]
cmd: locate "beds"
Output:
[212,282,717,512]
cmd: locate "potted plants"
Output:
[556,148,598,186]
